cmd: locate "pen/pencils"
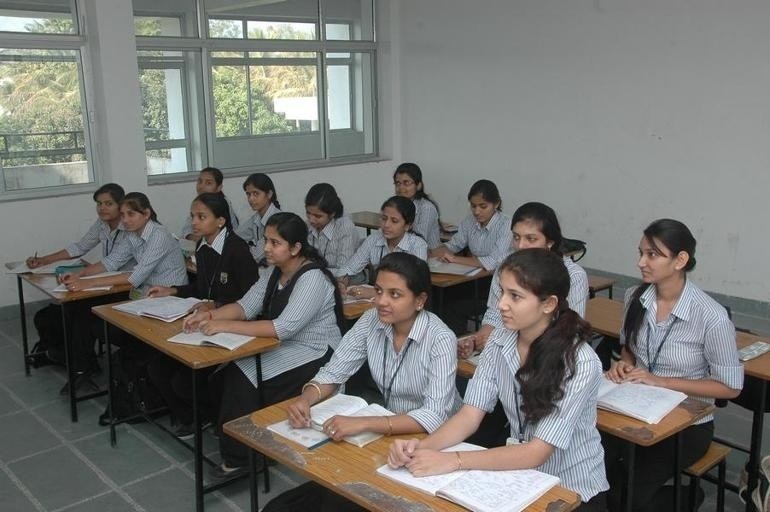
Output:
[34,251,38,259]
[342,273,348,284]
[186,308,197,333]
[171,233,180,240]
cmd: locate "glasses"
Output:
[393,179,418,187]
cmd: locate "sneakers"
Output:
[59,368,244,479]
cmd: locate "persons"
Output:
[605,216,744,512]
[254,249,465,512]
[23,159,592,479]
[385,246,614,512]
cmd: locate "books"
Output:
[596,371,690,425]
[374,441,561,512]
[6,258,84,279]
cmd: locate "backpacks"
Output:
[28,303,64,367]
